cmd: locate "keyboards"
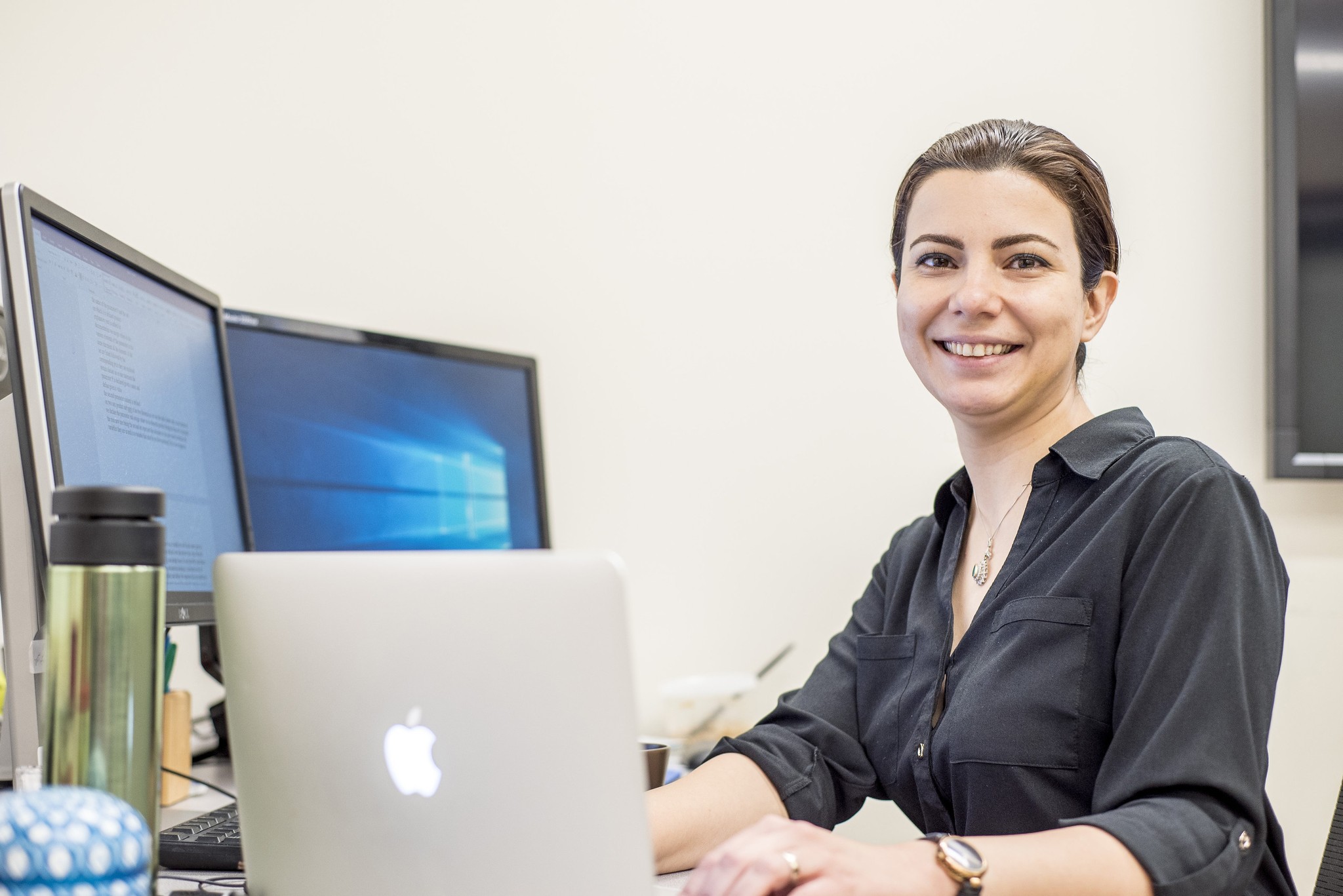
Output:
[158,802,245,872]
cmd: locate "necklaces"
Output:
[972,477,1032,588]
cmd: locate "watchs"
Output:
[916,833,987,896]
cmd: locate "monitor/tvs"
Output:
[0,180,548,836]
[1262,0,1343,483]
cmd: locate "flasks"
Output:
[39,484,167,893]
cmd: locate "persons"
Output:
[643,119,1299,896]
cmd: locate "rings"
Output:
[782,850,799,884]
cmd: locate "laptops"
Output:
[210,552,699,895]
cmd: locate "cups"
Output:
[643,741,670,792]
[158,692,191,807]
